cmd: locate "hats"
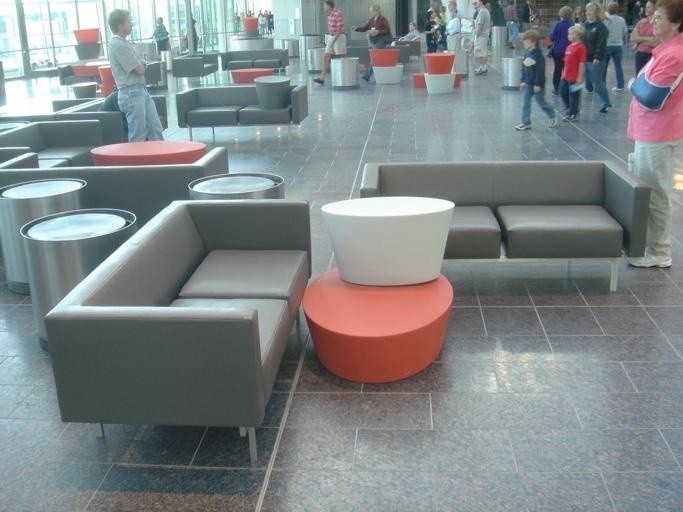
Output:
[559,6,572,18]
[608,3,617,12]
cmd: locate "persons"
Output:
[313,0,347,85]
[521,0,536,30]
[558,23,587,121]
[632,0,641,25]
[583,1,612,114]
[514,30,559,130]
[234,8,273,36]
[108,7,164,143]
[572,6,584,21]
[627,0,682,269]
[150,17,170,55]
[391,21,422,47]
[603,2,628,92]
[550,5,575,94]
[97,83,129,132]
[505,0,519,49]
[352,3,390,81]
[424,0,492,75]
[630,2,660,78]
[186,13,199,52]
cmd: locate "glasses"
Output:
[648,15,664,24]
[472,2,477,6]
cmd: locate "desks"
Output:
[0,177,138,354]
[187,171,286,199]
[491,25,507,48]
[502,56,521,88]
[280,30,360,90]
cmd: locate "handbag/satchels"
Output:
[544,48,552,58]
[628,18,646,52]
[381,32,393,43]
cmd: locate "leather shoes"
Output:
[313,78,323,84]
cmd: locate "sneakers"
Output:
[514,123,531,131]
[474,69,488,77]
[565,109,580,116]
[600,106,610,113]
[361,76,369,81]
[551,91,558,96]
[508,41,515,49]
[548,111,559,127]
[563,113,579,121]
[627,252,672,268]
[612,87,623,92]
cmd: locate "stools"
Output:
[369,47,403,85]
[97,66,116,97]
[230,68,274,83]
[70,81,96,98]
[302,263,454,384]
[254,76,290,108]
[322,196,454,287]
[412,52,462,95]
[73,28,103,60]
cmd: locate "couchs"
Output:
[0,94,230,231]
[43,199,312,466]
[344,39,420,63]
[360,160,652,293]
[58,64,102,86]
[145,61,162,91]
[221,49,289,82]
[172,53,218,82]
[176,83,308,143]
